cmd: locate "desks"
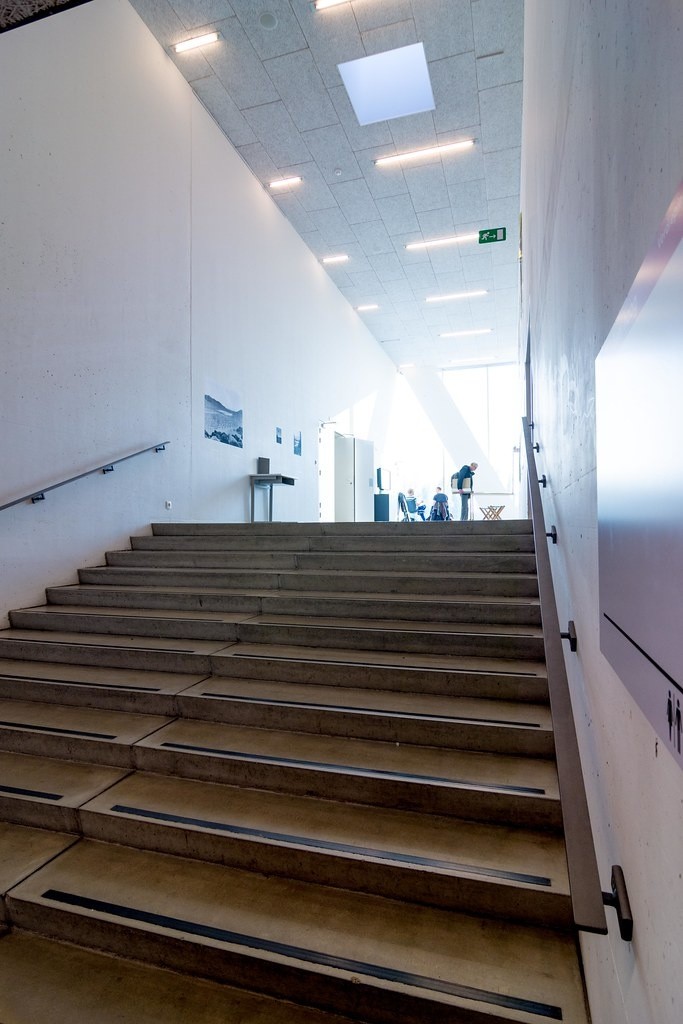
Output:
[479,505,504,520]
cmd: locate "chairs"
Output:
[397,493,419,523]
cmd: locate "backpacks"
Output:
[451,472,459,489]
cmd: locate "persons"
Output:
[426,487,451,521]
[457,462,478,521]
[407,488,426,522]
